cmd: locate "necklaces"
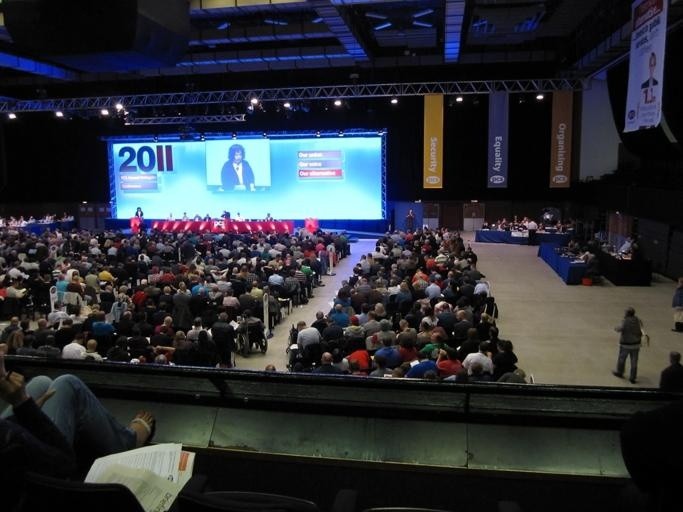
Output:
[233,160,241,170]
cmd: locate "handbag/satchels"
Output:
[640,334,649,347]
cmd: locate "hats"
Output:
[349,315,359,325]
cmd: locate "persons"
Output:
[641,52,658,89]
[0,370,153,512]
[221,144,256,192]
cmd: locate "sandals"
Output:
[129,411,155,446]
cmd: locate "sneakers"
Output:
[612,371,635,384]
